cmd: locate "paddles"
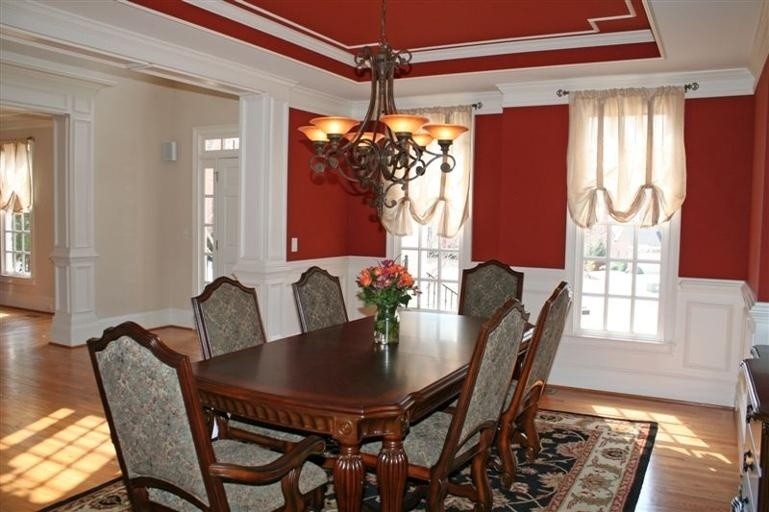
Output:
[37,406,658,512]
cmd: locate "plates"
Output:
[730,344,768,512]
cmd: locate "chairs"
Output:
[290,266,349,331]
[456,262,525,316]
[451,281,574,490]
[87,321,327,512]
[191,277,268,362]
[360,298,531,512]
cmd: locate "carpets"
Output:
[37,406,658,512]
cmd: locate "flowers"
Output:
[354,253,425,340]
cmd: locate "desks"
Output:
[189,312,534,512]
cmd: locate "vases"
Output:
[373,304,401,346]
[354,253,425,340]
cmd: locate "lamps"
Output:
[300,2,468,217]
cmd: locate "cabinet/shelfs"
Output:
[730,344,768,512]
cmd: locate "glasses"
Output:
[300,2,468,217]
